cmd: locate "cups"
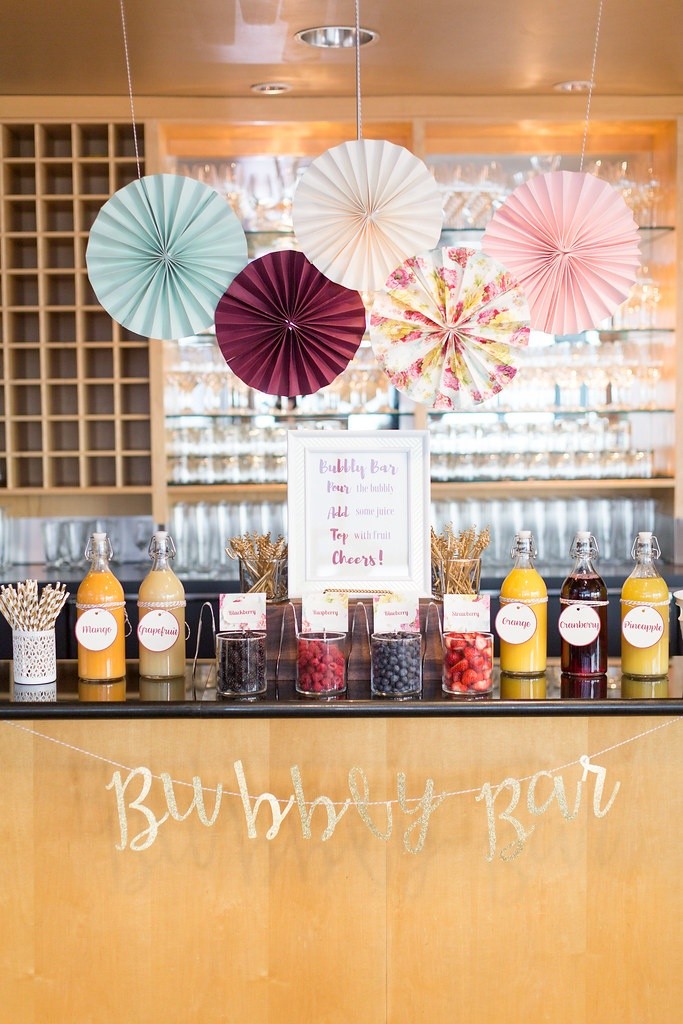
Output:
[239,557,288,604]
[370,631,422,696]
[430,557,482,602]
[295,632,347,697]
[217,632,266,697]
[442,631,495,695]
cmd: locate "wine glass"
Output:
[162,344,220,415]
[425,156,654,229]
[134,521,153,567]
[184,155,309,233]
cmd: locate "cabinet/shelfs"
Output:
[0,93,683,565]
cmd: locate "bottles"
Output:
[79,679,127,701]
[165,438,655,484]
[560,531,608,676]
[621,532,668,678]
[163,411,633,442]
[140,679,185,701]
[500,530,548,676]
[501,673,547,700]
[561,675,607,700]
[137,532,185,679]
[622,678,669,699]
[76,533,125,681]
[171,497,664,564]
